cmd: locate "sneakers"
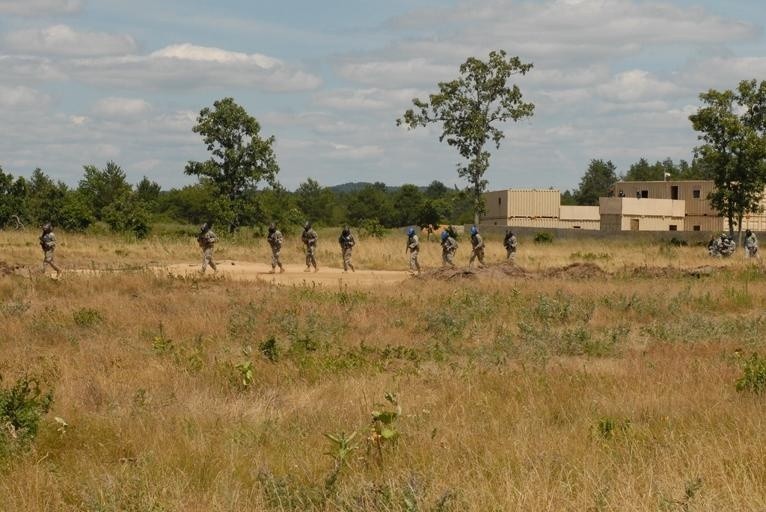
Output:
[195,266,220,277]
[341,266,357,276]
[302,264,321,274]
[267,266,287,276]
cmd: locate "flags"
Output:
[665,172,671,178]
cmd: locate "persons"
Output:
[338,225,357,274]
[708,228,760,260]
[635,189,642,198]
[438,230,459,269]
[502,229,518,260]
[608,189,615,197]
[468,226,489,267]
[618,188,625,197]
[39,222,64,280]
[406,228,422,277]
[196,222,221,275]
[300,222,321,273]
[266,223,286,274]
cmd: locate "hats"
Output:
[505,229,512,237]
[343,226,351,234]
[440,231,450,240]
[303,220,311,230]
[269,223,278,232]
[199,222,209,233]
[406,227,416,237]
[470,227,479,235]
